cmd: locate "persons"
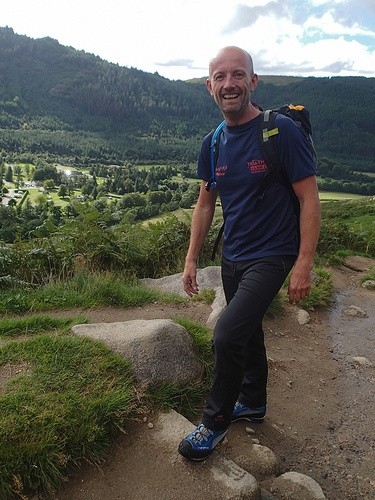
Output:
[177,45,321,462]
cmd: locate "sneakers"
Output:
[230,400,266,423]
[178,422,229,461]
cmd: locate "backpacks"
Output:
[204,104,318,193]
